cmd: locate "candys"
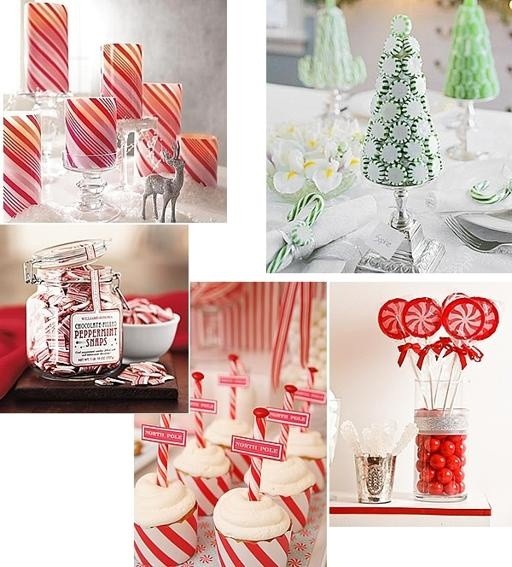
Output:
[26,263,175,385]
[378,292,499,496]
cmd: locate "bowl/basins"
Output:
[121,310,180,366]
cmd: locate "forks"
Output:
[443,215,511,256]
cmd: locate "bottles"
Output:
[19,243,124,381]
[414,406,468,503]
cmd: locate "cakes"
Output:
[134,354,327,567]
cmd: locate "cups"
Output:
[353,455,395,504]
